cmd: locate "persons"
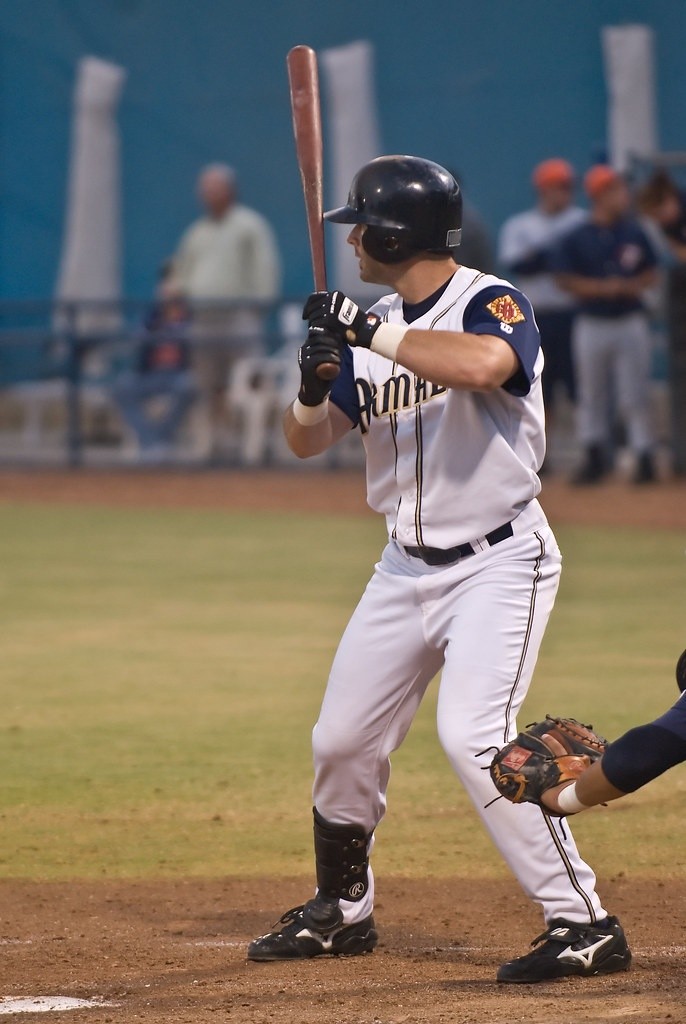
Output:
[247,158,686,982]
[134,154,686,493]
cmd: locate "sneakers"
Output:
[247,904,380,961]
[496,916,632,983]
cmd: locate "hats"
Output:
[534,157,578,188]
[585,164,620,195]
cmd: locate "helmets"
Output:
[323,155,463,266]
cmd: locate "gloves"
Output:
[301,290,409,363]
[293,327,344,427]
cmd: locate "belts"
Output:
[402,522,514,566]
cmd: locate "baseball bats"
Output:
[285,43,345,379]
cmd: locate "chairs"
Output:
[223,336,329,470]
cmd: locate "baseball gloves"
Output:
[489,713,614,819]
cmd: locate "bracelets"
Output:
[369,322,410,359]
[558,781,590,813]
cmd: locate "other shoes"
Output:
[569,443,613,487]
[630,452,654,486]
